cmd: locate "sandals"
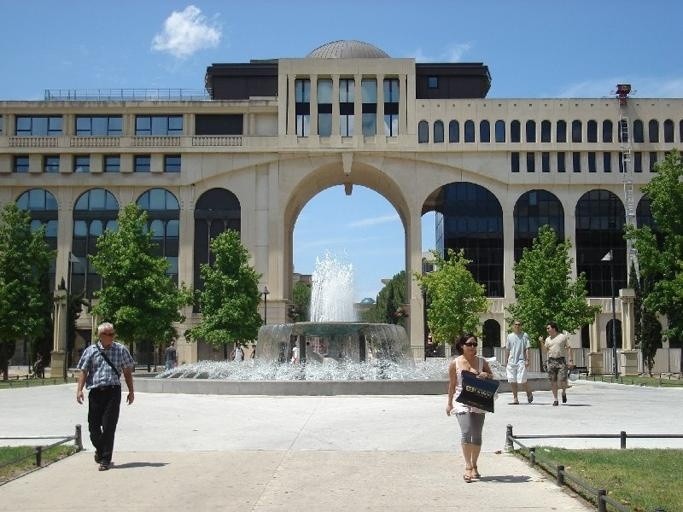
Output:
[464,466,479,482]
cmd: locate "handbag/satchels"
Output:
[568,368,577,381]
[456,370,500,413]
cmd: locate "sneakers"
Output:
[95,450,109,470]
[508,399,519,404]
[553,401,558,405]
[562,393,566,402]
[528,392,534,403]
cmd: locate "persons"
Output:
[444,333,494,484]
[30,351,44,380]
[290,345,299,365]
[75,321,136,472]
[305,342,313,361]
[228,342,244,361]
[163,341,177,372]
[540,321,574,406]
[250,344,255,358]
[504,320,534,405]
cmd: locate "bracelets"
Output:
[569,359,573,362]
[526,359,529,361]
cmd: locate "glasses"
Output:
[464,343,478,347]
